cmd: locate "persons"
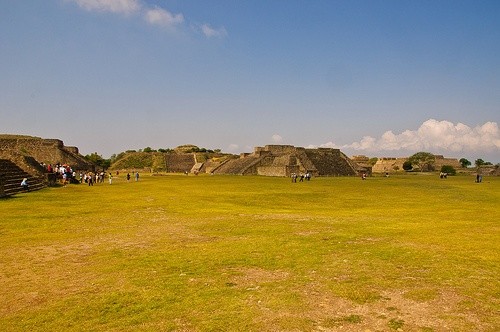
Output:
[304,172,312,181]
[298,175,304,182]
[108,172,113,185]
[126,172,131,180]
[439,171,444,178]
[116,171,119,175]
[134,171,139,182]
[443,172,448,178]
[184,168,190,176]
[291,172,297,183]
[361,171,368,179]
[194,169,199,175]
[40,161,107,186]
[474,171,483,183]
[20,177,29,190]
